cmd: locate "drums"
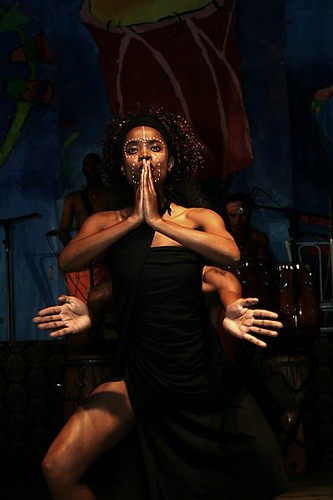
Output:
[55,348,114,433]
[261,348,310,480]
[58,247,108,326]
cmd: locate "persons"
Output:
[56,153,110,246]
[30,263,284,350]
[224,193,283,317]
[41,105,294,499]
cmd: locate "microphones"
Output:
[239,186,260,212]
